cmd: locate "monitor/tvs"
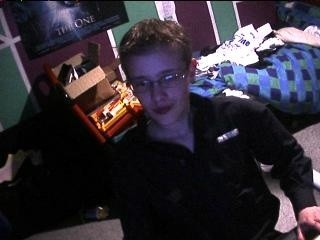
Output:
[10,0,129,61]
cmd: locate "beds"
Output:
[191,9,320,134]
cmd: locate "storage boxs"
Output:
[50,41,122,110]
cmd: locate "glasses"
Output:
[126,66,190,93]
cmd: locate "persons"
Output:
[82,19,320,239]
